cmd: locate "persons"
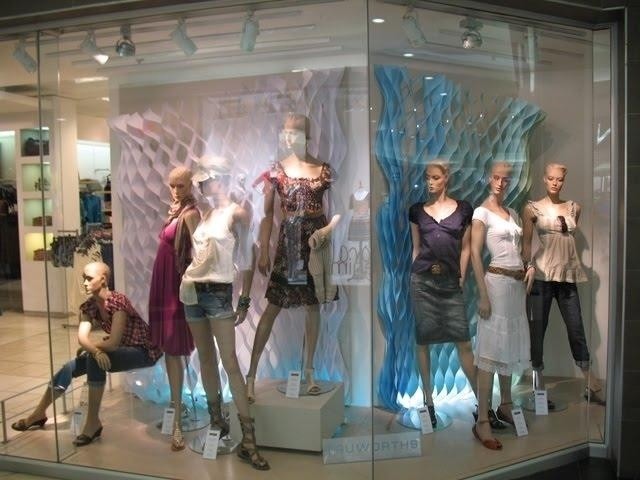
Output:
[246,114,341,404]
[470,162,531,451]
[521,162,607,408]
[11,262,163,447]
[408,159,505,429]
[149,166,204,453]
[179,155,270,470]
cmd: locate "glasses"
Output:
[558,214,569,235]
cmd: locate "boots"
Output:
[235,413,270,470]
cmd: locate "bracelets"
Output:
[94,349,105,357]
[525,261,536,271]
[238,296,251,312]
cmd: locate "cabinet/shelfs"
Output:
[13,124,86,317]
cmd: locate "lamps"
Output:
[398,1,485,51]
[11,5,265,77]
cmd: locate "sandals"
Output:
[156,401,189,428]
[172,421,185,450]
[201,394,230,450]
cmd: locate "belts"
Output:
[488,266,526,280]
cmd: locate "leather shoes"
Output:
[497,402,529,429]
[472,421,503,450]
[71,427,103,445]
[12,417,48,431]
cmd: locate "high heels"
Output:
[472,404,505,430]
[245,374,256,404]
[425,402,437,425]
[585,386,606,406]
[532,391,556,409]
[302,367,322,394]
